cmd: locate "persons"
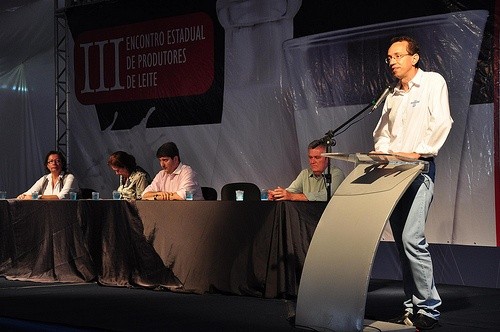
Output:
[108,151,152,201]
[16,150,81,201]
[369,35,454,329]
[267,140,346,204]
[138,141,205,201]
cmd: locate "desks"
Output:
[0,191,329,300]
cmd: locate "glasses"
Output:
[47,159,61,165]
[386,54,416,64]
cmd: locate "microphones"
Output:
[370,82,394,113]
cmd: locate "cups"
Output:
[261,191,268,201]
[236,190,244,201]
[32,191,38,199]
[0,191,7,200]
[186,191,193,201]
[92,192,99,199]
[113,191,120,200]
[70,192,76,200]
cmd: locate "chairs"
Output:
[221,182,262,201]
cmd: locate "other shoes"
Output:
[391,312,438,332]
